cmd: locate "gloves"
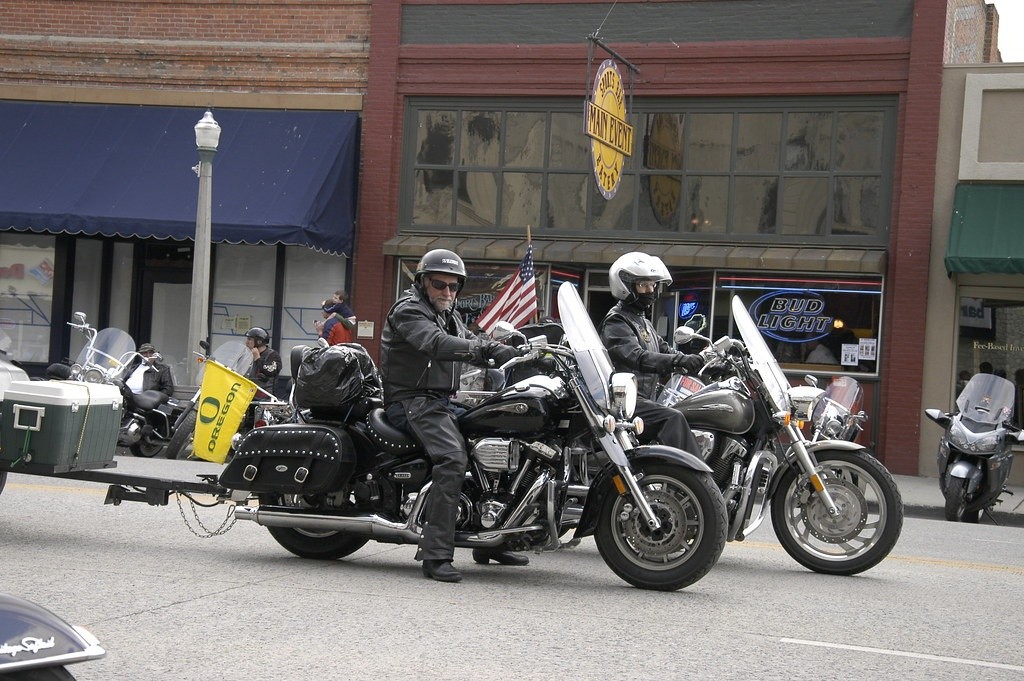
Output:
[673,354,704,376]
[483,340,530,370]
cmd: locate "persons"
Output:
[379,247,530,581]
[191,325,283,461]
[313,290,358,347]
[124,342,173,396]
[804,319,876,373]
[596,252,746,527]
[960,362,1024,430]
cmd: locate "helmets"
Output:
[609,252,673,301]
[245,327,269,344]
[414,249,467,298]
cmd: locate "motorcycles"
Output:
[165,337,309,462]
[216,279,730,592]
[923,372,1024,522]
[520,294,913,575]
[46,310,187,457]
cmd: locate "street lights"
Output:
[190,108,220,392]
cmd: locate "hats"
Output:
[138,343,155,353]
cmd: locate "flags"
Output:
[475,244,537,341]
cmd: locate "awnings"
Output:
[944,182,1024,279]
[0,97,361,260]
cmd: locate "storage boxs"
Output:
[218,422,355,498]
[1,380,123,465]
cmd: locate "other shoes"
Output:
[313,320,317,324]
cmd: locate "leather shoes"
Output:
[422,560,462,582]
[472,549,529,566]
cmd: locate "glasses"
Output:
[429,278,462,292]
[635,283,657,289]
